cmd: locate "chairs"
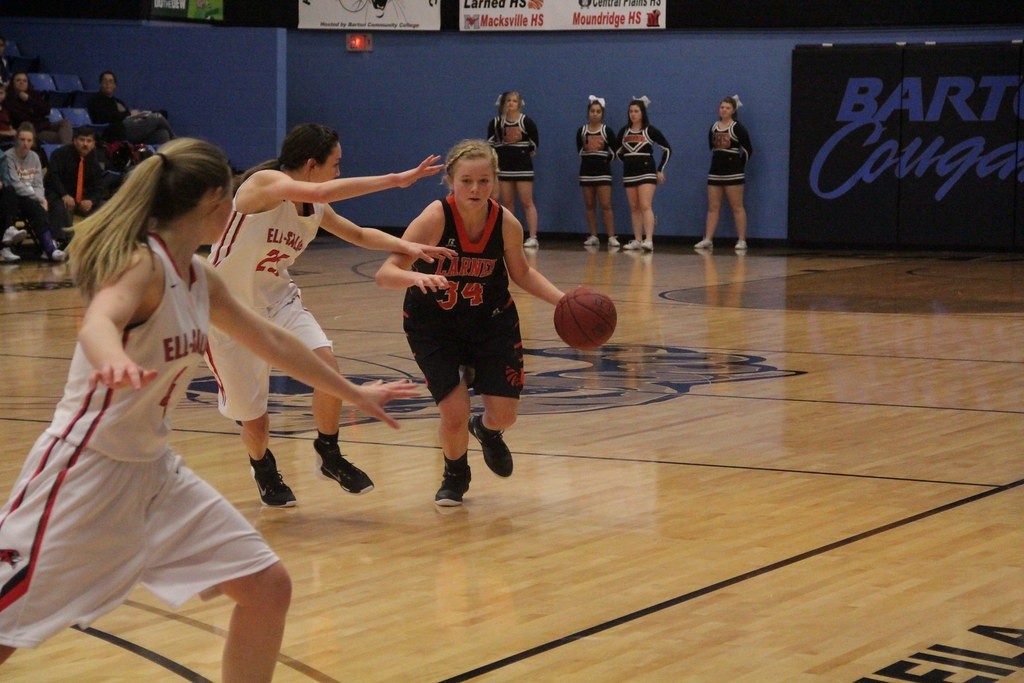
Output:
[0,39,162,259]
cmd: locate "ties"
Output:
[76,156,83,203]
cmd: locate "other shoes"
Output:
[52,250,65,261]
[41,251,48,259]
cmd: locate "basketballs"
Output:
[554,287,617,351]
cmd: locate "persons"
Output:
[576,93,620,246]
[0,139,422,682]
[375,140,563,505]
[693,93,752,250]
[199,123,459,508]
[88,70,176,144]
[616,95,672,251]
[486,91,539,247]
[0,36,108,263]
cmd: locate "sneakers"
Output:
[313,438,374,494]
[624,240,643,249]
[468,413,513,477]
[608,237,620,246]
[608,246,619,253]
[2,226,26,245]
[642,239,653,251]
[0,248,19,261]
[435,463,471,506]
[524,239,539,246]
[623,249,644,256]
[524,247,539,255]
[695,249,712,255]
[585,245,599,252]
[640,251,653,261]
[584,236,600,245]
[735,240,746,249]
[735,249,746,256]
[250,448,296,507]
[695,239,713,248]
